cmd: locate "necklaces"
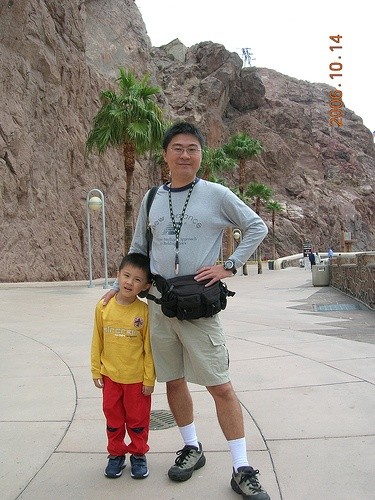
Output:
[168,179,196,273]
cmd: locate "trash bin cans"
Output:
[268,261,274,270]
[312,264,330,286]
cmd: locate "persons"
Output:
[98,121,269,500]
[309,247,334,267]
[90,252,156,478]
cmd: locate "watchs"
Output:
[224,259,237,275]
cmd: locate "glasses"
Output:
[168,145,200,155]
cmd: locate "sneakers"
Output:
[130,455,149,478]
[167,442,206,481]
[104,453,128,478]
[230,466,270,500]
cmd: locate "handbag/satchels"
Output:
[146,274,236,321]
